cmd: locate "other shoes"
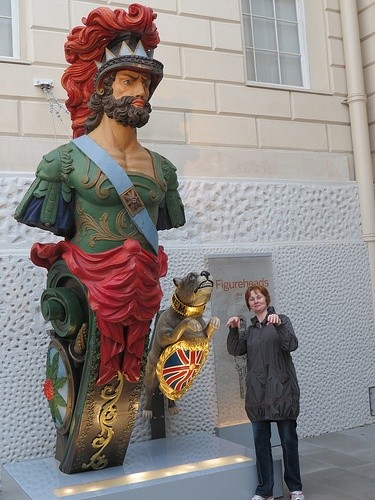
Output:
[251,494,274,500]
[290,491,304,500]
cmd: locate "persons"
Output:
[16,3,186,254]
[226,286,305,500]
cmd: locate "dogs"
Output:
[142,270,221,419]
